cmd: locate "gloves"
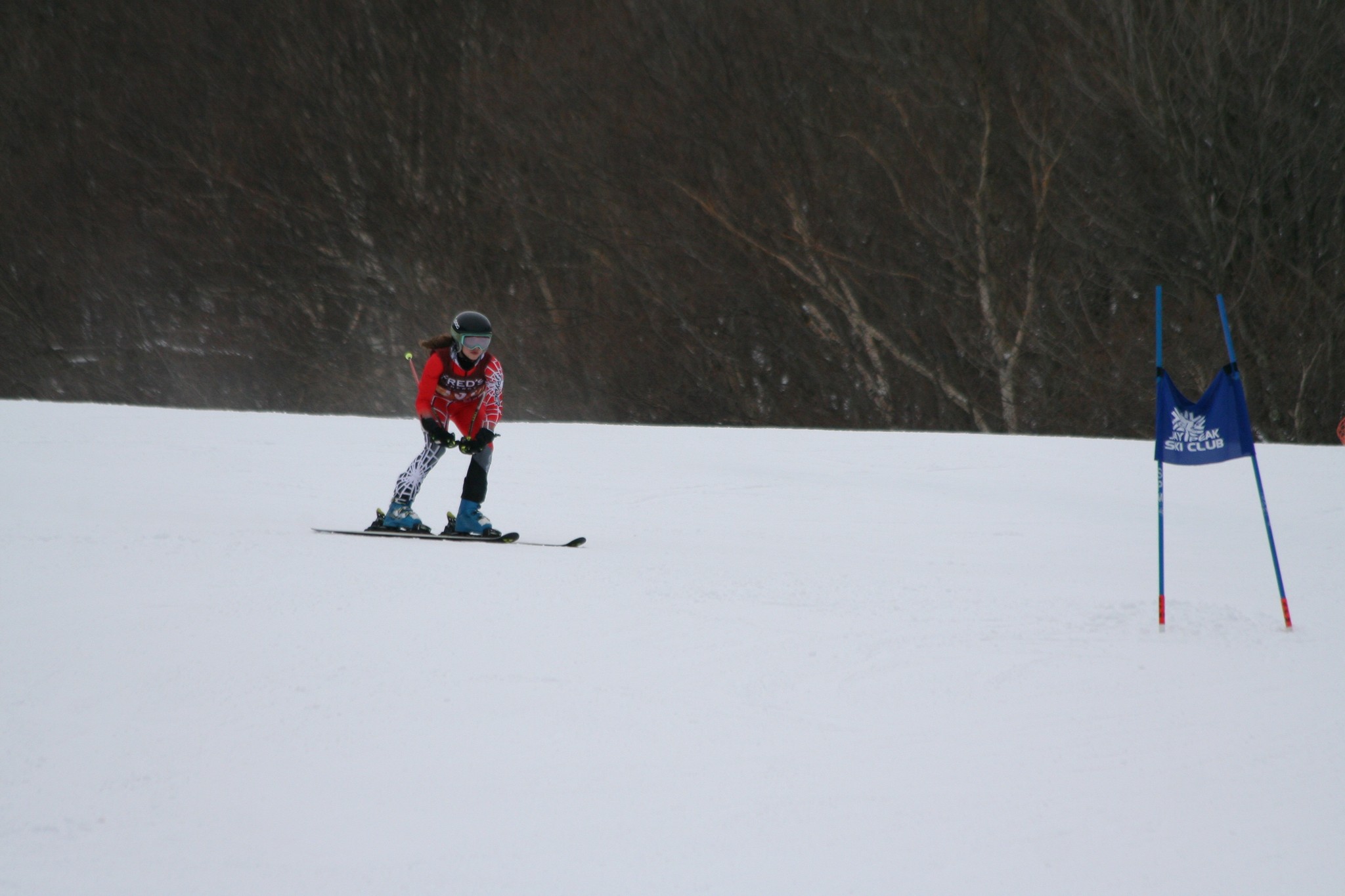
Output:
[422,418,459,448]
[460,429,495,454]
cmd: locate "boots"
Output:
[383,502,423,529]
[454,499,491,535]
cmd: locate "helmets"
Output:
[451,310,493,352]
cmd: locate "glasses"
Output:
[451,330,491,350]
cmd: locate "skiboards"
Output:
[310,507,587,548]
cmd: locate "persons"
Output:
[381,311,504,537]
